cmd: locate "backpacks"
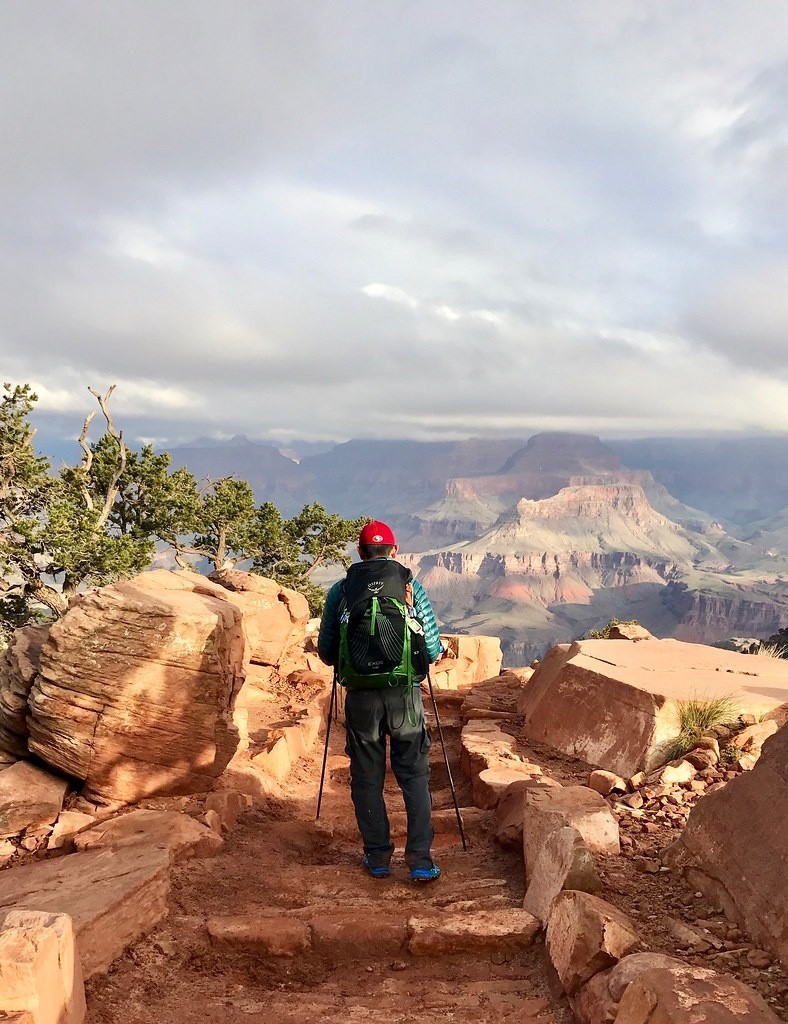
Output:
[336,568,419,727]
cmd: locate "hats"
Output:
[358,520,397,545]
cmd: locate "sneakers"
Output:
[411,863,441,881]
[362,854,391,878]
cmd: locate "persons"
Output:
[318,520,441,882]
